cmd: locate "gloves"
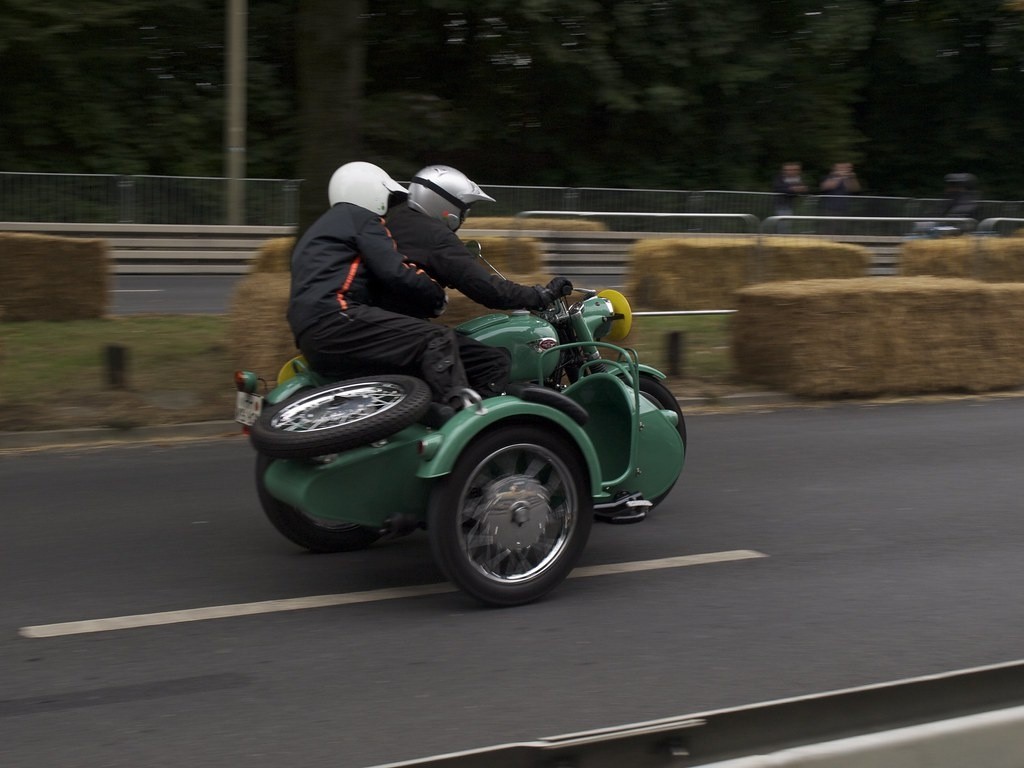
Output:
[544,277,573,300]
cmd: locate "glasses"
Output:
[411,177,471,221]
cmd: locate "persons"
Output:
[818,161,862,236]
[285,161,573,400]
[772,162,811,234]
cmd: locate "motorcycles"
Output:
[235,283,689,607]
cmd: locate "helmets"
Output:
[327,161,412,217]
[407,165,496,232]
